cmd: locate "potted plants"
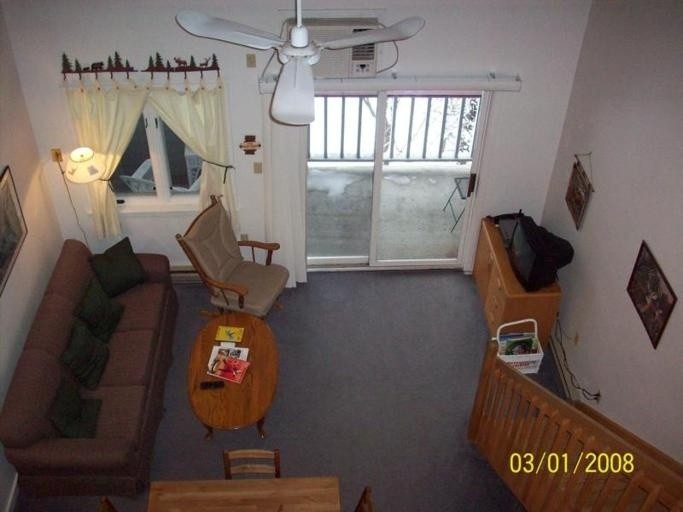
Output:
[0,165,29,297]
[565,160,594,231]
[625,240,677,350]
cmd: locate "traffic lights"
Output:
[87,236,150,298]
[72,286,125,333]
[59,321,109,390]
[45,380,102,438]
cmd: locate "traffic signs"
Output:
[50,148,62,163]
[595,389,603,405]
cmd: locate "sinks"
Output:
[6,239,179,500]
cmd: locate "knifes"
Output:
[283,17,376,81]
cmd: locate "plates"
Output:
[473,212,562,352]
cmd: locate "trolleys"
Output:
[175,0,426,127]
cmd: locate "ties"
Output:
[507,216,575,293]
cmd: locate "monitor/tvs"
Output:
[206,345,249,384]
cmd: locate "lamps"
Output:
[98,494,116,512]
[442,176,469,233]
[175,195,290,319]
[120,159,201,194]
[356,487,373,511]
[224,449,281,478]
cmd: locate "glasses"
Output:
[63,147,107,184]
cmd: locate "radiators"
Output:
[200,381,225,389]
